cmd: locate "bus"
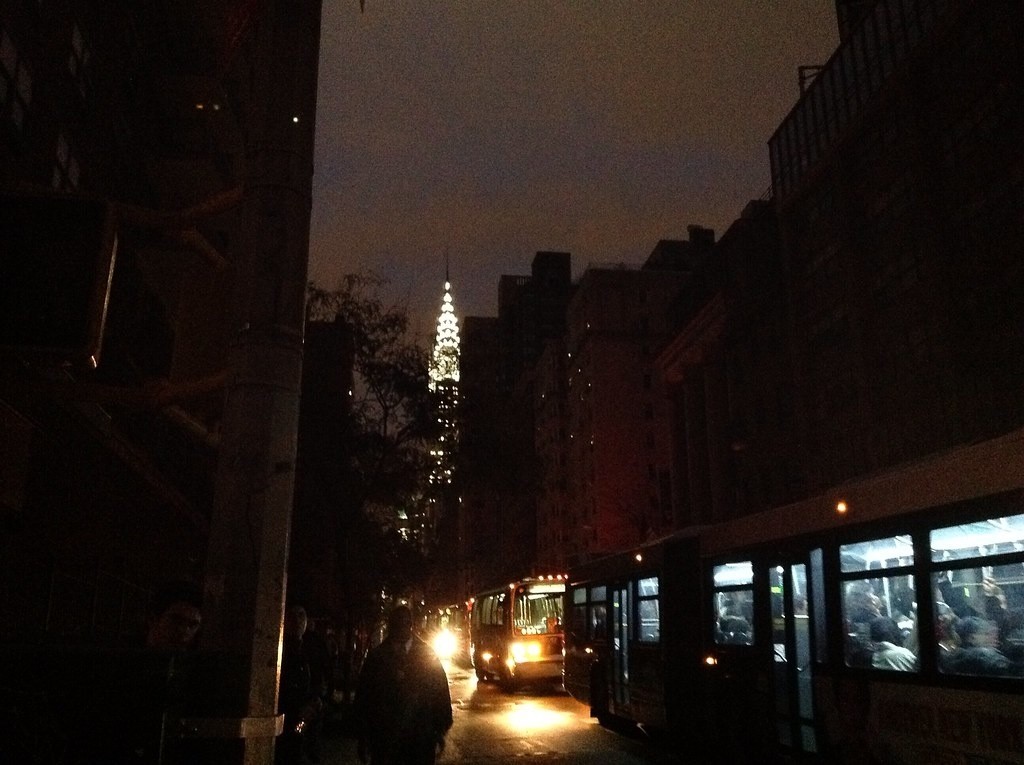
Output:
[562,421,1024,765]
[428,571,569,690]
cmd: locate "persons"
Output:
[107,581,206,765]
[353,606,448,765]
[308,614,363,654]
[593,563,1024,676]
[275,599,334,765]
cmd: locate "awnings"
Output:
[569,524,710,588]
